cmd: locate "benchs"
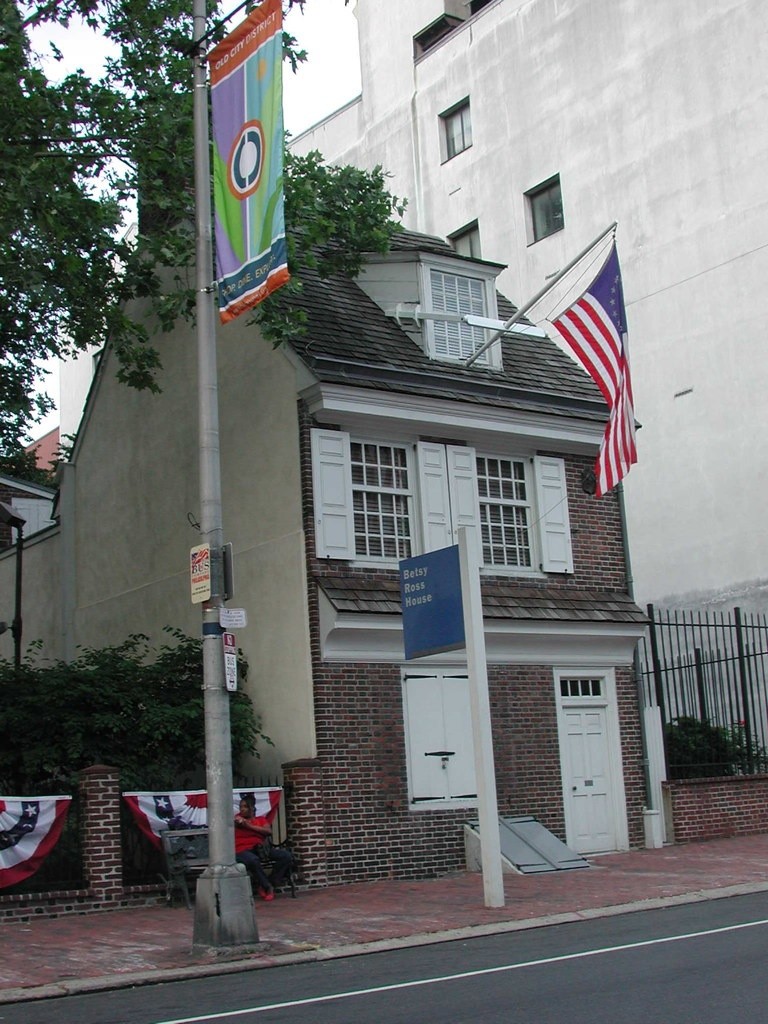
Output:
[157,825,299,912]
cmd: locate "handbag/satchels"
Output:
[253,840,270,862]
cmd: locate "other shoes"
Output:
[258,888,266,897]
[264,893,274,901]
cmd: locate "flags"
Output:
[543,240,638,498]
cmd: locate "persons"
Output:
[235,796,291,900]
